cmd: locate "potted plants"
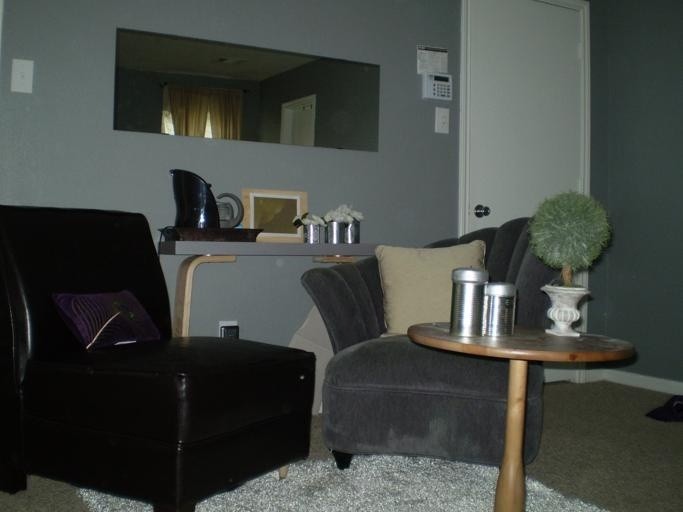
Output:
[530,192,611,336]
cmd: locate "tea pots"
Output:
[217,192,244,228]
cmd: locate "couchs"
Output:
[299,218,563,472]
[1,206,316,512]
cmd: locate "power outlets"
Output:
[219,324,241,339]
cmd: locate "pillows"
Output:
[58,286,158,354]
[375,239,488,336]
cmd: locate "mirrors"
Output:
[114,27,380,153]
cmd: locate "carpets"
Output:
[0,376,683,512]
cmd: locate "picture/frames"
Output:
[243,186,309,242]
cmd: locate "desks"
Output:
[407,318,637,511]
[153,223,377,337]
